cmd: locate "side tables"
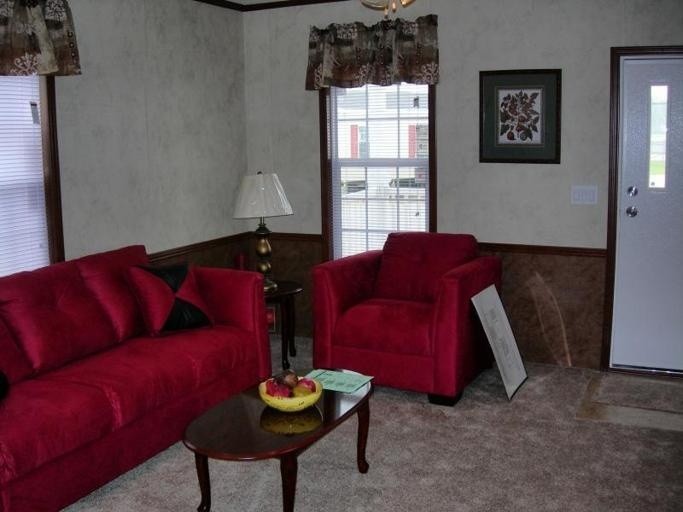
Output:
[257,281,310,368]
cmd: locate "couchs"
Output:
[1,242,274,512]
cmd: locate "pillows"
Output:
[10,262,195,373]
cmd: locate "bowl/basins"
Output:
[258,376,324,413]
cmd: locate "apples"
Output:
[266,369,315,398]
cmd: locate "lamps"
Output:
[231,171,294,290]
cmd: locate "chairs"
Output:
[314,231,493,408]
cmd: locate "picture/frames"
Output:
[479,68,562,164]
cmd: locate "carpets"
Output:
[591,372,683,414]
[63,334,682,512]
